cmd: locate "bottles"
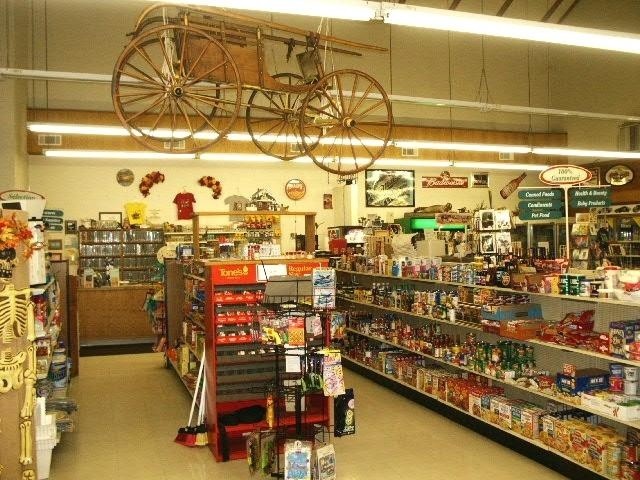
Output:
[476,248,527,288]
[379,257,476,286]
[617,257,640,269]
[370,282,530,334]
[399,323,460,364]
[614,243,640,255]
[341,334,426,375]
[344,310,407,344]
[460,333,534,379]
[619,223,639,240]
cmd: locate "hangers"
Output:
[180,185,190,194]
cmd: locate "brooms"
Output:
[174,341,210,448]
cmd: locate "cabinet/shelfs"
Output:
[0,210,640,479]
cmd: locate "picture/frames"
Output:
[364,168,415,208]
[471,172,490,188]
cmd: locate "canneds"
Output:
[607,442,640,480]
[451,333,536,380]
[559,273,603,298]
[411,288,459,322]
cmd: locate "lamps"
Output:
[0,1,639,172]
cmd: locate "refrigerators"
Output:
[513,216,578,259]
